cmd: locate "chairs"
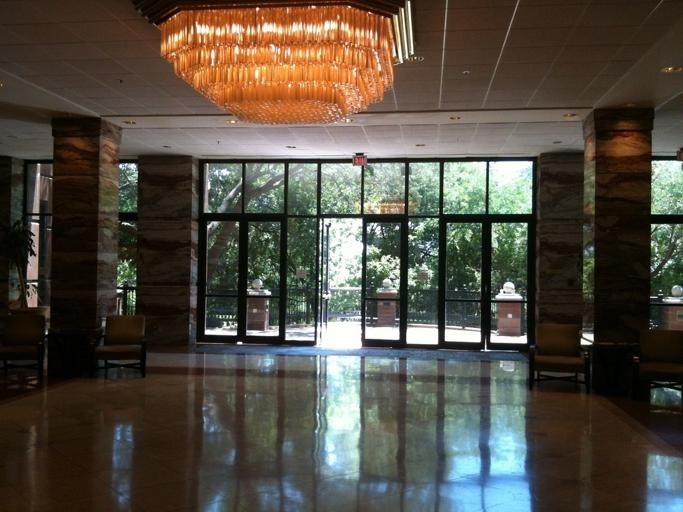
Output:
[526,324,591,395]
[91,313,149,381]
[0,313,48,383]
[632,329,682,403]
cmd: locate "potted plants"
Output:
[1,217,49,332]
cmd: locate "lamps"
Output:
[135,0,413,125]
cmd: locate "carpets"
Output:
[185,340,526,362]
